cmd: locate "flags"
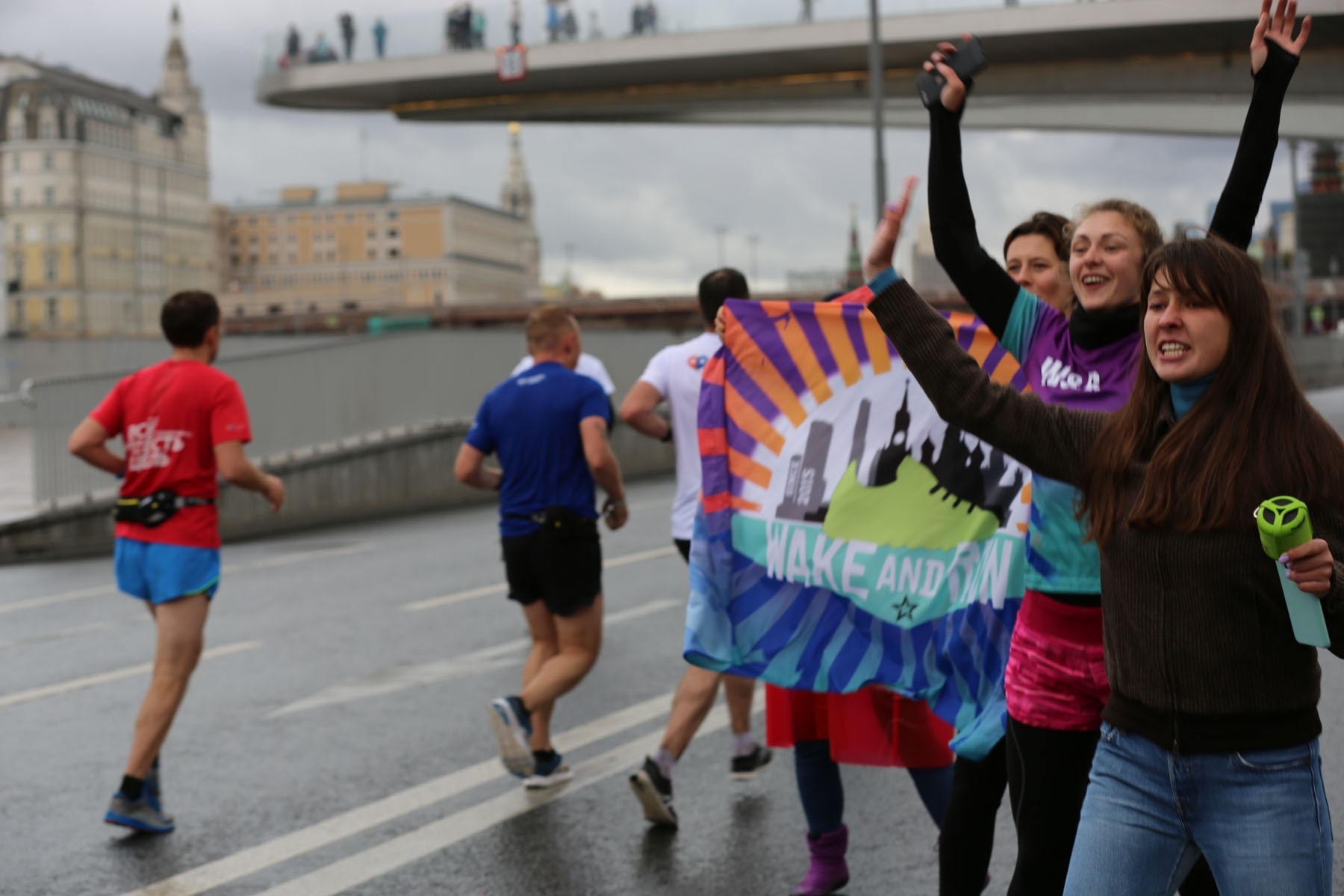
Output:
[682,300,1035,761]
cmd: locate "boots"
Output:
[788,824,850,896]
[937,833,990,892]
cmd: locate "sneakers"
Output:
[494,693,536,778]
[731,741,774,779]
[524,749,574,789]
[105,773,175,835]
[628,755,680,826]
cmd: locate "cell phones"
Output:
[1275,560,1332,648]
[915,37,986,109]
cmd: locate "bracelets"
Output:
[660,428,673,442]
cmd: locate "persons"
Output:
[509,345,617,436]
[454,302,630,789]
[68,290,285,833]
[622,0,1344,896]
[619,268,773,828]
